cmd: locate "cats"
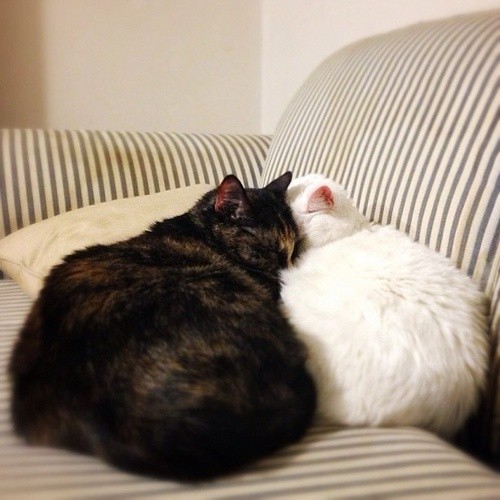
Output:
[8,169,318,489]
[278,174,492,438]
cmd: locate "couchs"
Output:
[0,8,500,499]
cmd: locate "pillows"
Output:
[0,184,219,301]
